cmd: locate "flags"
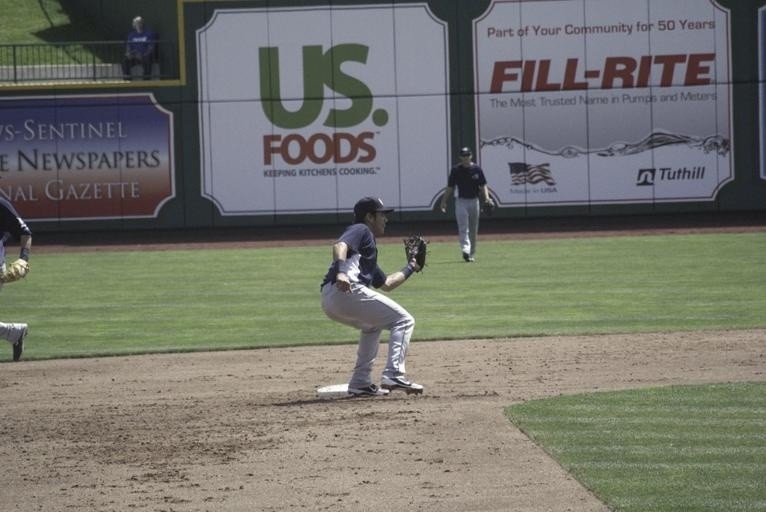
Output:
[508,162,557,186]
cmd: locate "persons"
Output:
[0,194,32,361]
[320,194,428,396]
[121,16,157,80]
[440,147,494,262]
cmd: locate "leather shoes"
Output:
[14,322,27,361]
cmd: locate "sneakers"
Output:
[380,375,423,394]
[347,383,390,397]
[463,251,470,262]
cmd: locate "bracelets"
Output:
[399,263,415,279]
[19,247,29,262]
[335,260,348,274]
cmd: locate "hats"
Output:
[459,146,471,156]
[353,197,394,218]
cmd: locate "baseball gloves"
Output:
[481,198,497,212]
[0,256,26,283]
[403,234,426,271]
